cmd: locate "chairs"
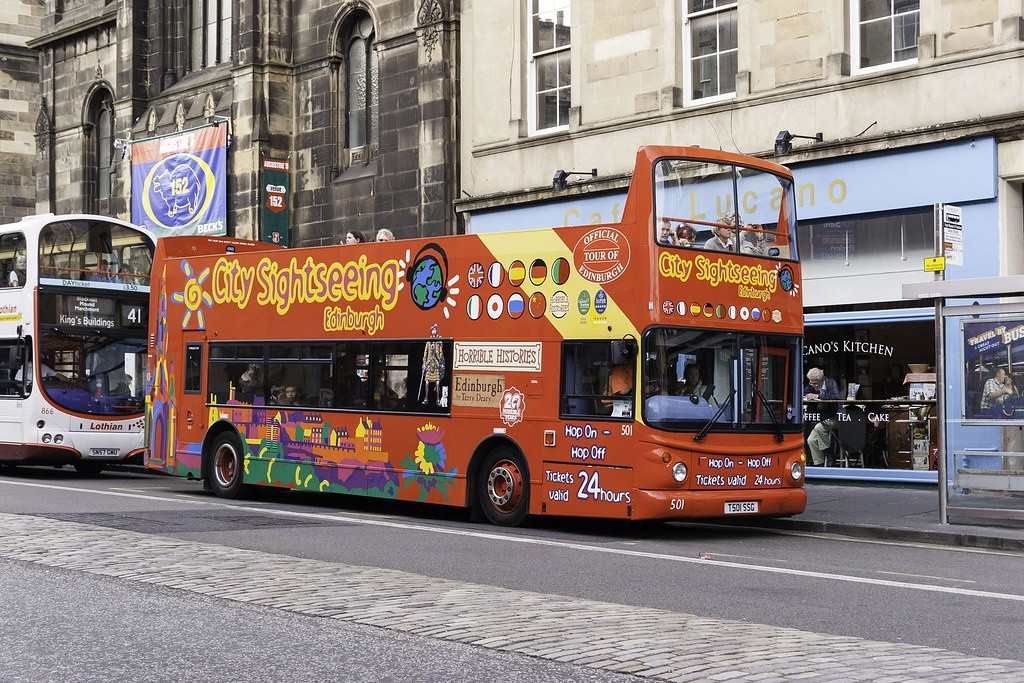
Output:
[80,268,93,281]
[59,273,71,279]
[39,268,56,279]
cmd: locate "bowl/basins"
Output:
[908,364,931,373]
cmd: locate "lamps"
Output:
[661,143,702,176]
[552,168,598,192]
[773,130,823,157]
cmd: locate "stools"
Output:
[824,420,864,468]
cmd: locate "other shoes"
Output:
[826,453,835,467]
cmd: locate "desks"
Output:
[754,397,937,405]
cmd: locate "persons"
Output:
[9,255,26,287]
[677,363,712,396]
[239,362,264,396]
[346,231,366,245]
[376,228,395,242]
[804,368,844,466]
[89,259,138,285]
[601,345,660,408]
[365,363,397,400]
[703,213,774,258]
[285,384,312,407]
[657,220,697,248]
[15,349,69,391]
[980,368,1019,411]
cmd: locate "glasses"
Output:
[810,380,821,385]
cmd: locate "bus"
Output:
[0,211,159,478]
[140,142,809,528]
